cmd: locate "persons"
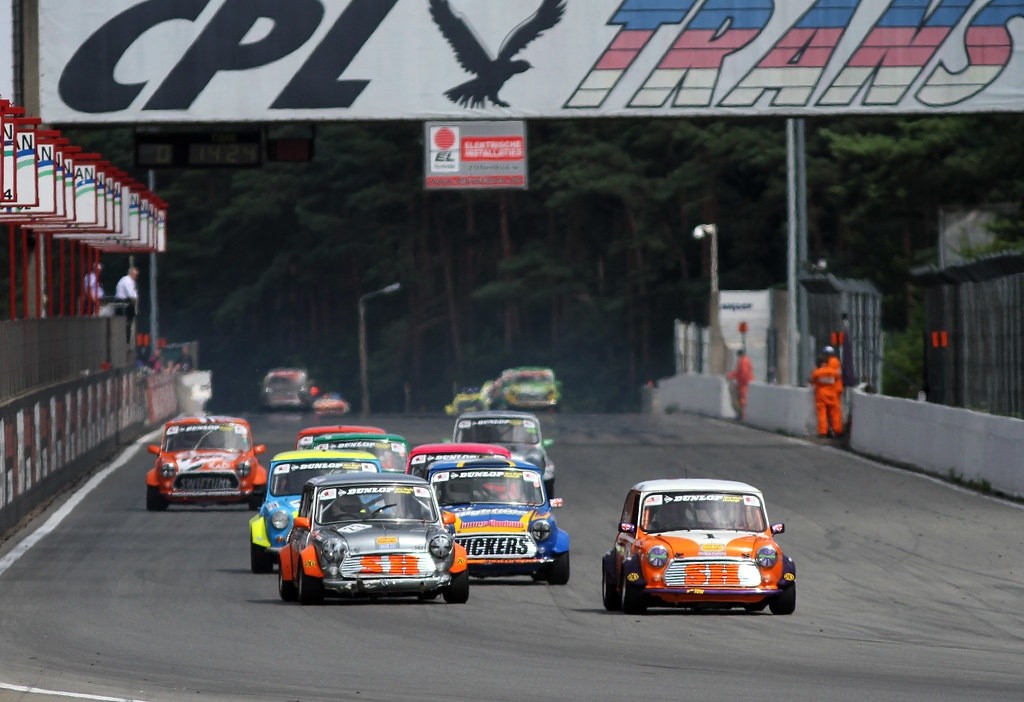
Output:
[112,267,140,343]
[81,261,105,316]
[809,345,847,439]
[133,345,199,377]
[726,343,754,422]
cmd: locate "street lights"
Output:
[355,282,403,417]
[695,222,725,377]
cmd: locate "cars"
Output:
[146,414,271,513]
[263,367,311,410]
[452,410,558,493]
[425,458,571,586]
[406,441,515,487]
[599,476,797,618]
[502,364,562,411]
[249,450,384,576]
[310,432,412,480]
[445,392,492,420]
[280,473,470,604]
[313,391,351,419]
[295,424,393,453]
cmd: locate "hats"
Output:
[822,345,835,355]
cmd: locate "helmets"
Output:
[332,494,361,518]
[446,480,474,502]
